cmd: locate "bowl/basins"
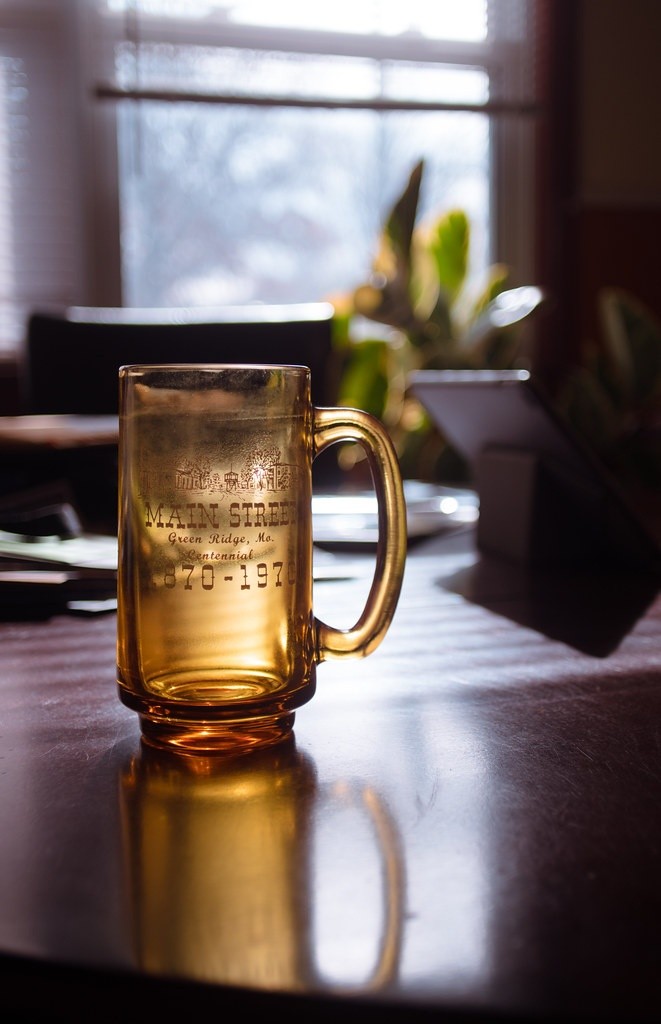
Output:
[408,374,558,462]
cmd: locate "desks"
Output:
[2,480,661,1024]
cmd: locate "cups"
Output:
[113,361,410,759]
